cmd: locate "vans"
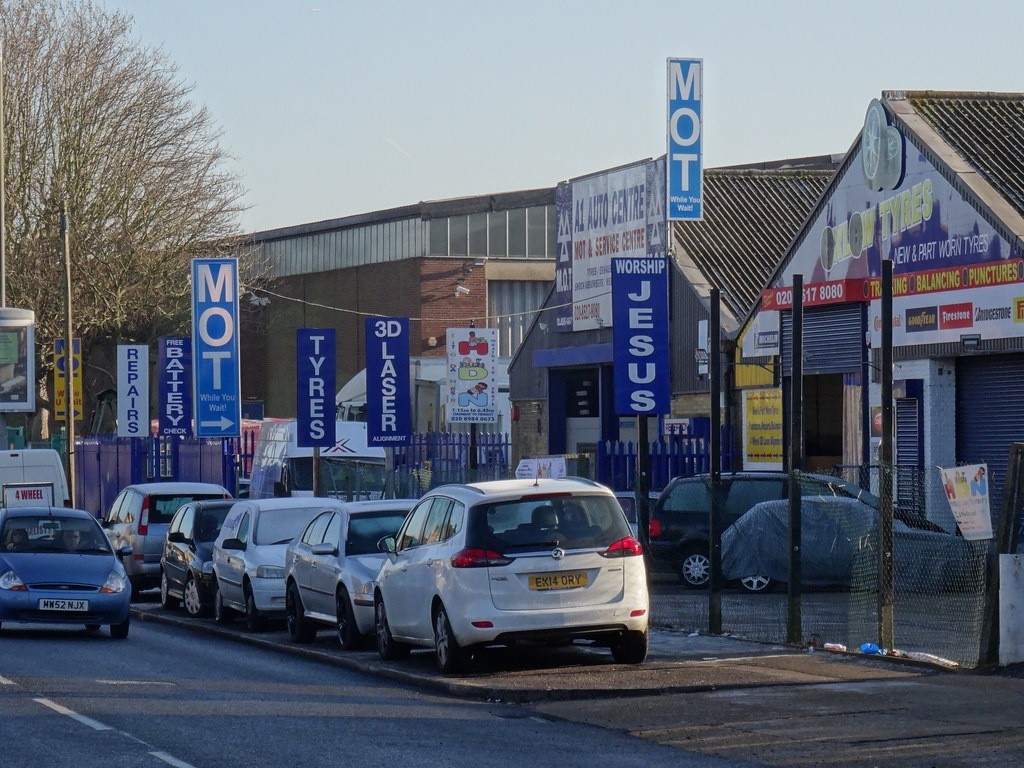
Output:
[249,419,388,503]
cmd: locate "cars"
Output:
[159,499,236,620]
[212,498,346,632]
[0,507,133,640]
[97,484,233,601]
[720,496,971,595]
[372,476,649,677]
[285,499,420,649]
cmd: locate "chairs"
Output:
[200,514,220,542]
[528,505,567,544]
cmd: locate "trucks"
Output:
[0,450,73,540]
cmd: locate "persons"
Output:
[62,530,106,552]
[5,528,29,552]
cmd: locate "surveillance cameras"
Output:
[456,286,470,295]
[428,340,437,348]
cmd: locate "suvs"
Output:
[650,469,951,591]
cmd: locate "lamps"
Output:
[473,257,488,269]
[694,349,709,365]
[960,333,981,350]
